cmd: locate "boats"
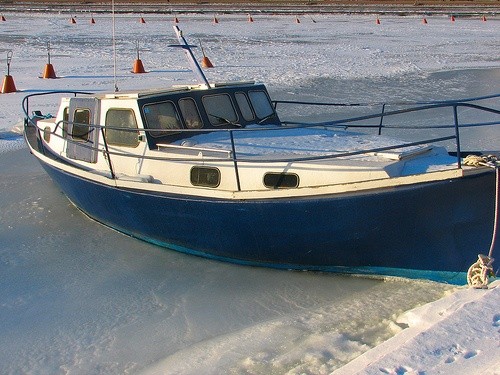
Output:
[20,78,500,286]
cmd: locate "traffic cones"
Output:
[1,11,488,94]
